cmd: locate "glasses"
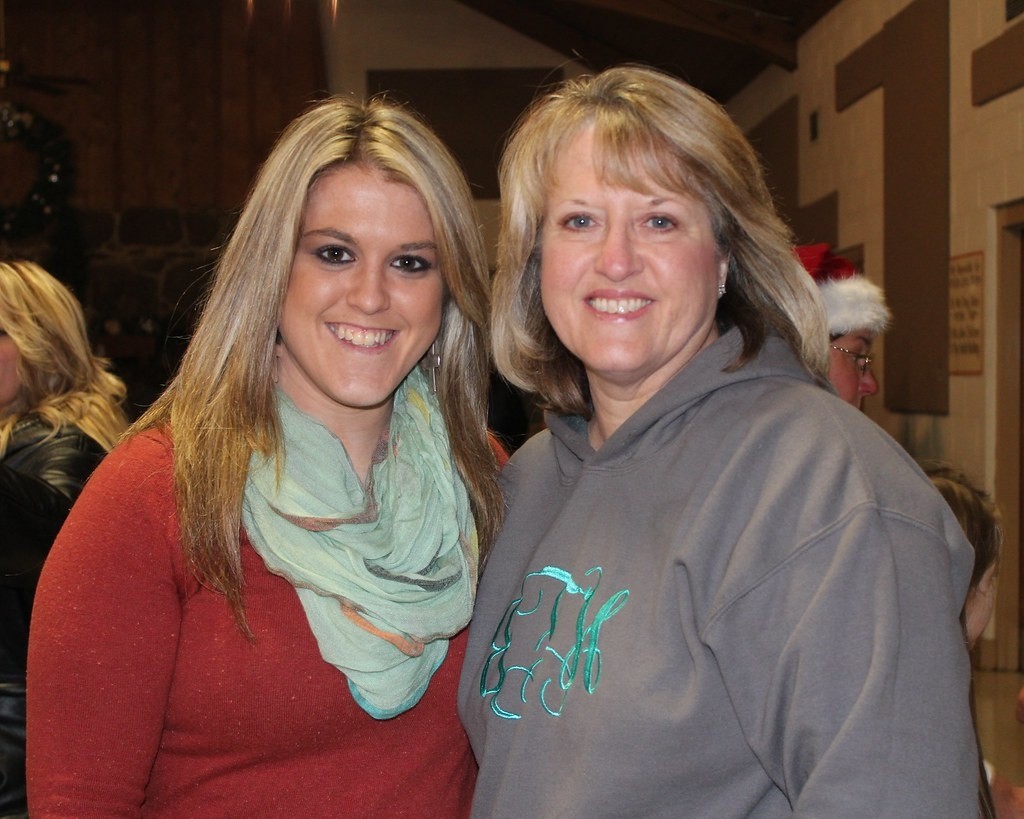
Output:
[827,343,877,377]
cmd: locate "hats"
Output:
[793,242,894,336]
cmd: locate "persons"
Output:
[459,68,979,819]
[26,97,508,819]
[791,243,890,409]
[0,261,131,819]
[921,467,1002,819]
